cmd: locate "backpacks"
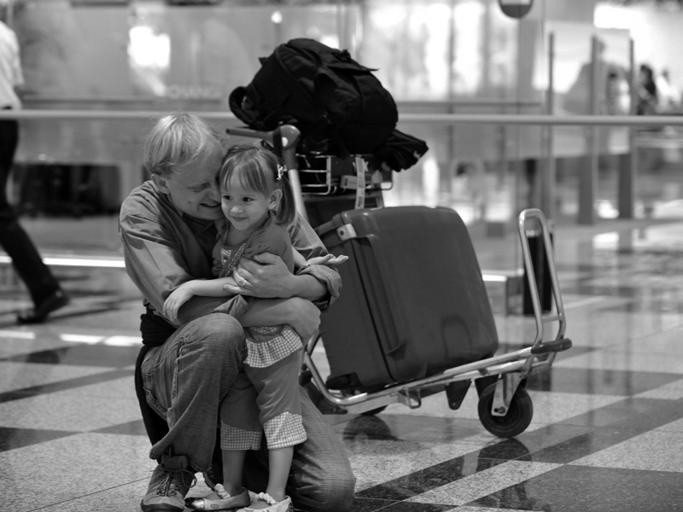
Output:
[228,38,399,145]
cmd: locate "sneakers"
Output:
[141,446,197,512]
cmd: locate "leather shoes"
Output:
[17,289,69,324]
[191,483,250,511]
[235,492,294,512]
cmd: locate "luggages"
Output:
[287,147,393,195]
[306,204,499,390]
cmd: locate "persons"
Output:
[0,20,69,325]
[163,146,305,512]
[117,113,356,512]
[565,37,681,118]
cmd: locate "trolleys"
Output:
[225,118,572,440]
